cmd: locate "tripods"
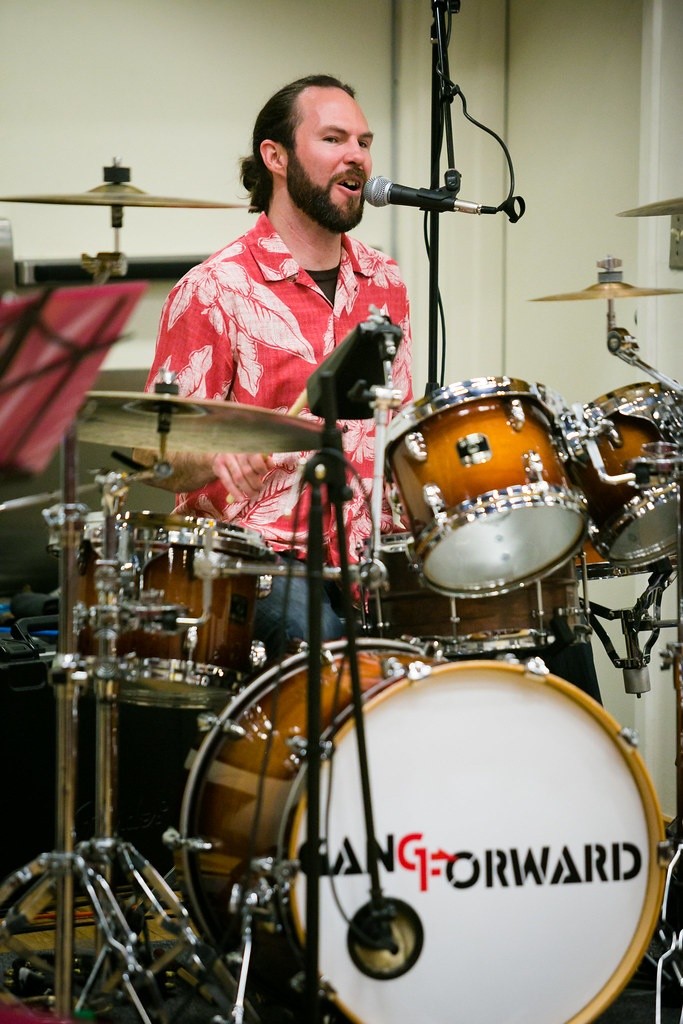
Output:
[0,414,263,1024]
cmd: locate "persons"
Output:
[124,73,414,668]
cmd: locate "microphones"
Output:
[359,915,396,949]
[362,176,496,215]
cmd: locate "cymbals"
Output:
[615,197,682,218]
[73,388,324,456]
[0,183,260,209]
[526,281,682,304]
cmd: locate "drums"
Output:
[177,635,668,1024]
[77,507,264,711]
[384,376,591,601]
[363,530,589,660]
[563,377,681,569]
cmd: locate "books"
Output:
[0,281,150,477]
